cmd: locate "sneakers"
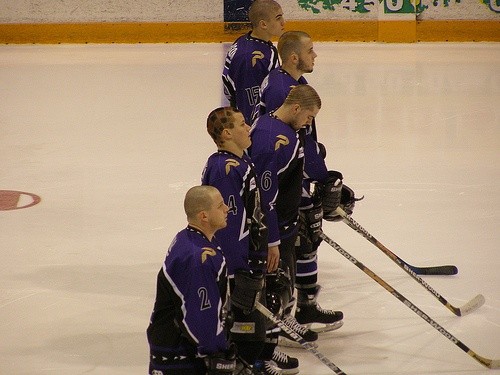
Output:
[277,297,318,347]
[296,285,344,334]
[259,351,299,372]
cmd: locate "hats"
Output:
[322,182,365,221]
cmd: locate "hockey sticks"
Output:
[409,258,458,278]
[335,206,486,318]
[252,299,349,375]
[316,226,500,371]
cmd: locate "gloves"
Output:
[305,206,323,241]
[205,353,257,375]
[320,169,343,208]
[232,268,278,315]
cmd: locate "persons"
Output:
[246,86,321,375]
[200,107,282,375]
[251,31,343,348]
[221,0,285,127]
[147,186,238,375]
